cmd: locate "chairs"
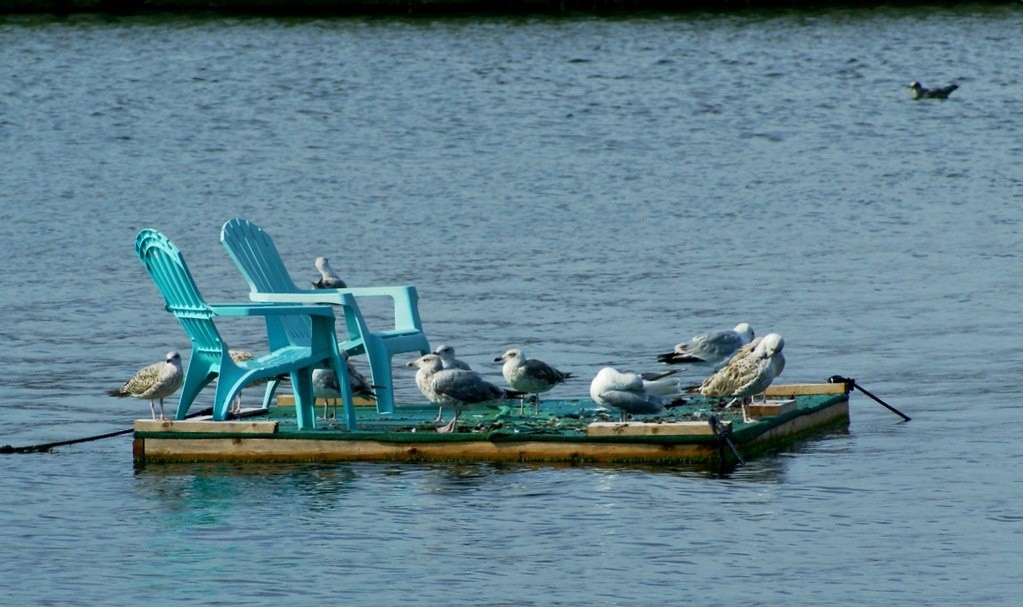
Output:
[220,218,430,415]
[133,228,358,431]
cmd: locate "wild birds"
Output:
[908,80,959,100]
[657,322,755,373]
[432,344,482,423]
[494,349,580,418]
[106,352,185,421]
[311,362,387,420]
[406,356,529,433]
[682,333,788,423]
[214,351,290,415]
[307,257,347,291]
[589,366,689,422]
